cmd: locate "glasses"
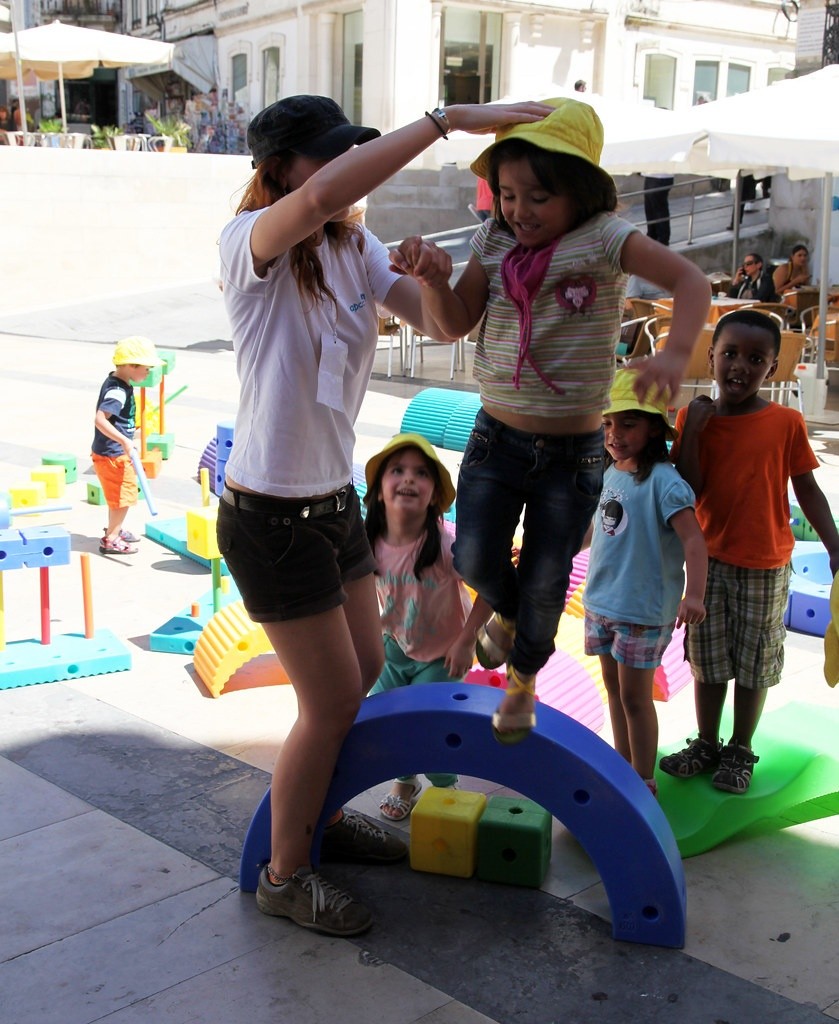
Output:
[745,259,758,266]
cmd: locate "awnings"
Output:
[125,35,216,102]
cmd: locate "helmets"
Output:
[112,336,167,366]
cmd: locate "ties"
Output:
[500,235,564,396]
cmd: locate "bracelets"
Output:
[425,111,449,141]
[432,108,451,134]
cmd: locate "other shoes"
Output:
[647,779,656,796]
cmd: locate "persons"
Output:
[575,79,588,92]
[730,252,775,303]
[643,175,675,245]
[660,311,839,792]
[510,368,707,793]
[624,273,671,309]
[215,95,556,937]
[363,435,491,821]
[773,245,812,296]
[92,336,167,554]
[389,97,711,738]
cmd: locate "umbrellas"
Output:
[0,19,177,133]
[447,63,839,379]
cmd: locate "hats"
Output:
[603,369,680,441]
[366,433,456,515]
[246,95,382,161]
[712,311,782,355]
[470,97,617,210]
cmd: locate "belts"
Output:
[222,486,352,520]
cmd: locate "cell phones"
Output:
[742,269,746,275]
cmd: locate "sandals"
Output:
[713,743,759,794]
[659,740,724,779]
[100,538,138,554]
[477,613,516,670]
[493,665,536,745]
[103,528,140,542]
[380,777,422,821]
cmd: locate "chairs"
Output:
[372,271,839,416]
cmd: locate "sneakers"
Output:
[256,863,374,935]
[320,809,409,862]
